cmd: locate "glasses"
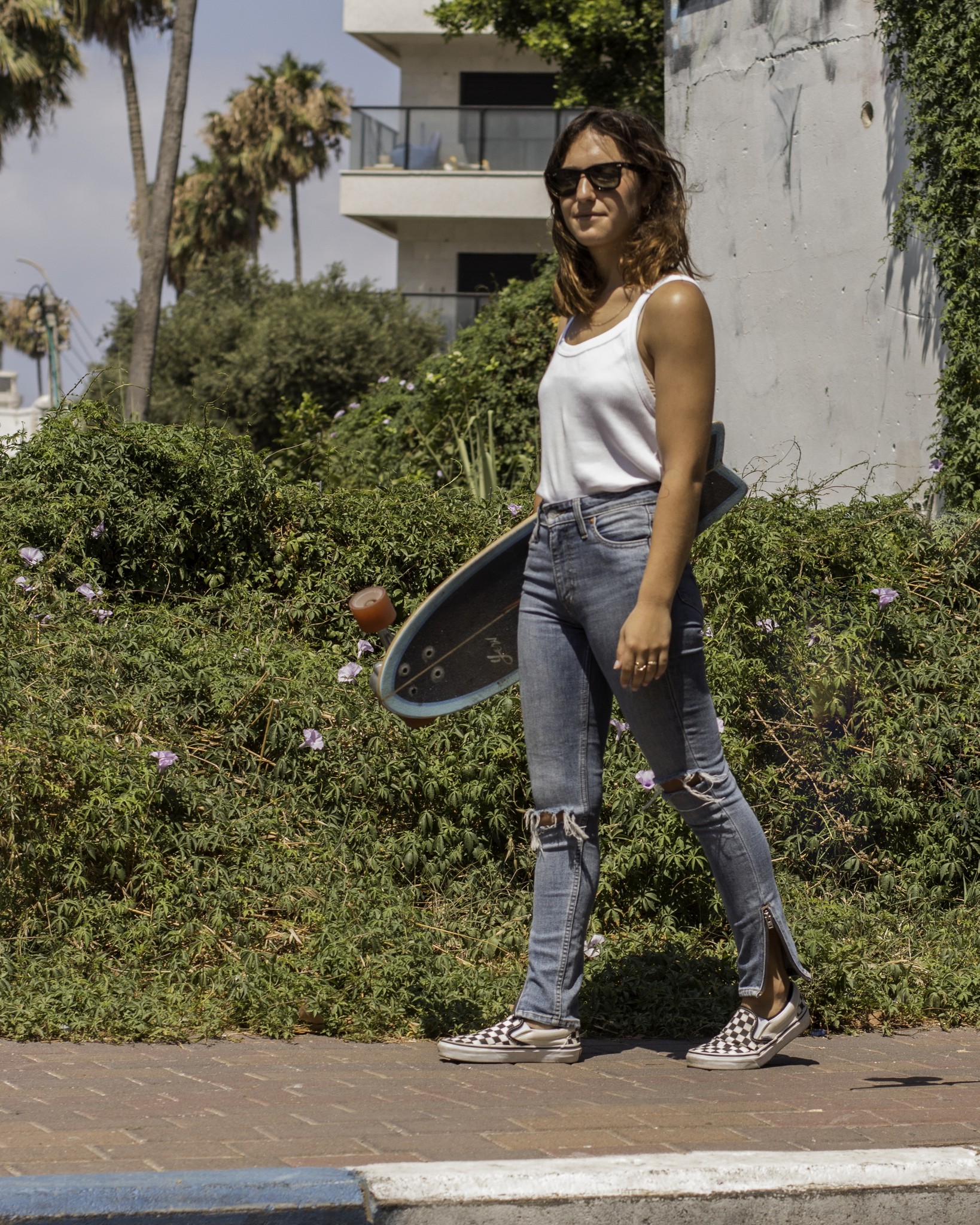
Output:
[544,162,651,198]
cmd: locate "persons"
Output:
[441,106,812,1065]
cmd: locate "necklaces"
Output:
[567,279,637,339]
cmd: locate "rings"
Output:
[635,662,648,671]
[646,661,657,665]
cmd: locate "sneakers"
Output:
[437,1013,584,1065]
[685,981,812,1071]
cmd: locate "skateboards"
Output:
[347,421,751,729]
